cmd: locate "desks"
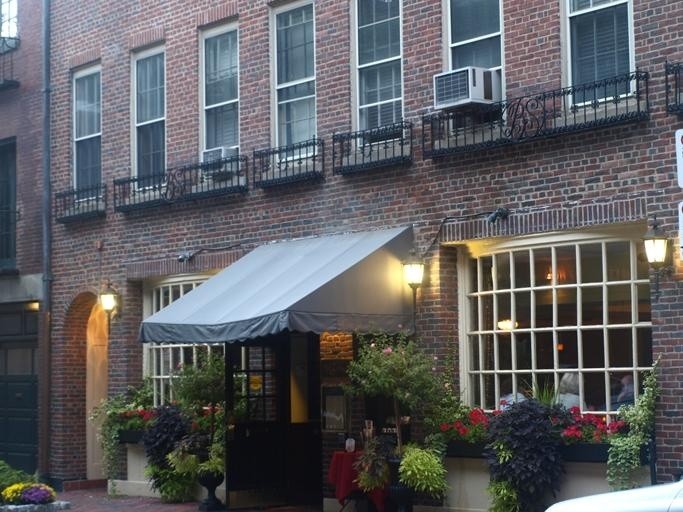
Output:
[333,451,387,512]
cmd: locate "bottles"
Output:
[345,438,356,453]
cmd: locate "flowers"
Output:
[425,404,632,447]
[85,397,251,431]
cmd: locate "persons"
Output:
[498,373,527,410]
[601,375,640,411]
[549,369,584,410]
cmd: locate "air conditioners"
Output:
[432,64,499,110]
[200,146,237,175]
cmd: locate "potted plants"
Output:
[347,331,447,512]
[173,347,230,512]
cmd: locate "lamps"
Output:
[643,217,669,301]
[99,281,118,335]
[401,246,425,330]
